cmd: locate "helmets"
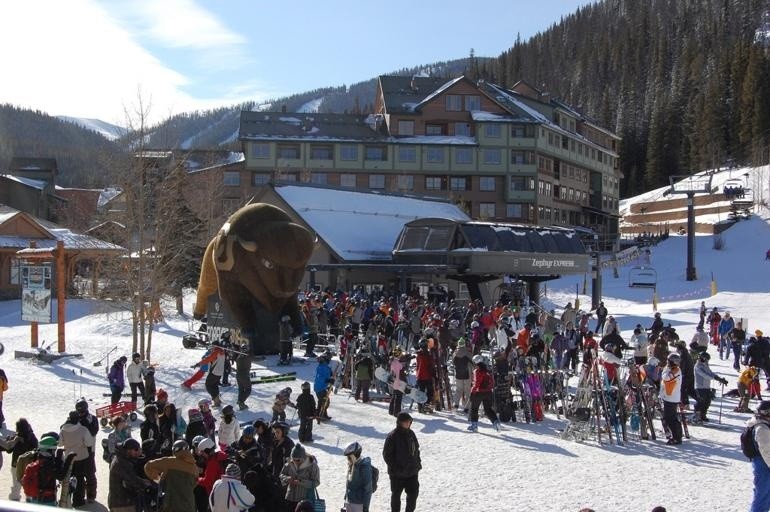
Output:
[120,352,155,373]
[142,438,160,455]
[69,411,81,420]
[191,435,216,453]
[171,439,190,454]
[242,425,257,439]
[211,331,249,352]
[222,405,233,415]
[756,400,770,415]
[189,398,212,419]
[37,431,60,451]
[76,401,88,411]
[344,442,362,456]
[277,287,763,374]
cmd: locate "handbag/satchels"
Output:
[303,479,325,512]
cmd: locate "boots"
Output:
[466,421,479,431]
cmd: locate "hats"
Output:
[277,421,291,437]
[256,418,271,430]
[291,443,306,459]
[225,463,242,478]
[143,388,175,415]
[161,439,172,454]
[301,382,310,391]
[121,438,140,449]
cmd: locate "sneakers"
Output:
[236,400,249,411]
[87,496,95,503]
[667,437,682,445]
[222,383,232,387]
[72,499,86,507]
[493,420,500,429]
[212,395,223,407]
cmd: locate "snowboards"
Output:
[374,367,428,404]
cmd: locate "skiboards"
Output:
[60,456,77,507]
[334,336,391,395]
[489,338,571,425]
[103,393,142,397]
[251,371,297,383]
[316,361,340,424]
[430,344,453,412]
[560,357,690,445]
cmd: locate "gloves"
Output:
[66,453,77,461]
[57,449,65,457]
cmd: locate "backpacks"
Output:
[20,454,55,499]
[740,422,770,459]
[371,465,379,492]
[15,448,40,485]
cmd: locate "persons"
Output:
[578,506,594,512]
[634,230,657,248]
[724,184,746,200]
[739,398,770,512]
[650,504,668,512]
